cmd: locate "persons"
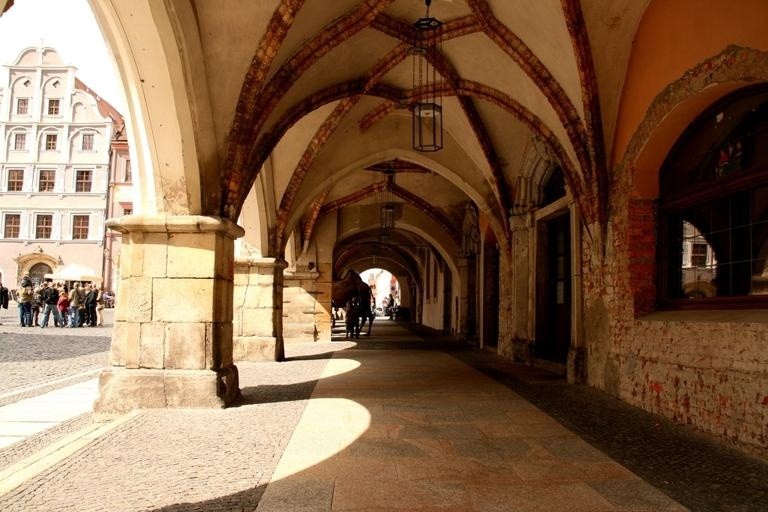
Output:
[16,270,106,329]
[0,272,9,326]
[344,282,399,339]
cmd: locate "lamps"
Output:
[370,243,379,266]
[380,164,398,234]
[378,228,392,250]
[408,0,449,160]
